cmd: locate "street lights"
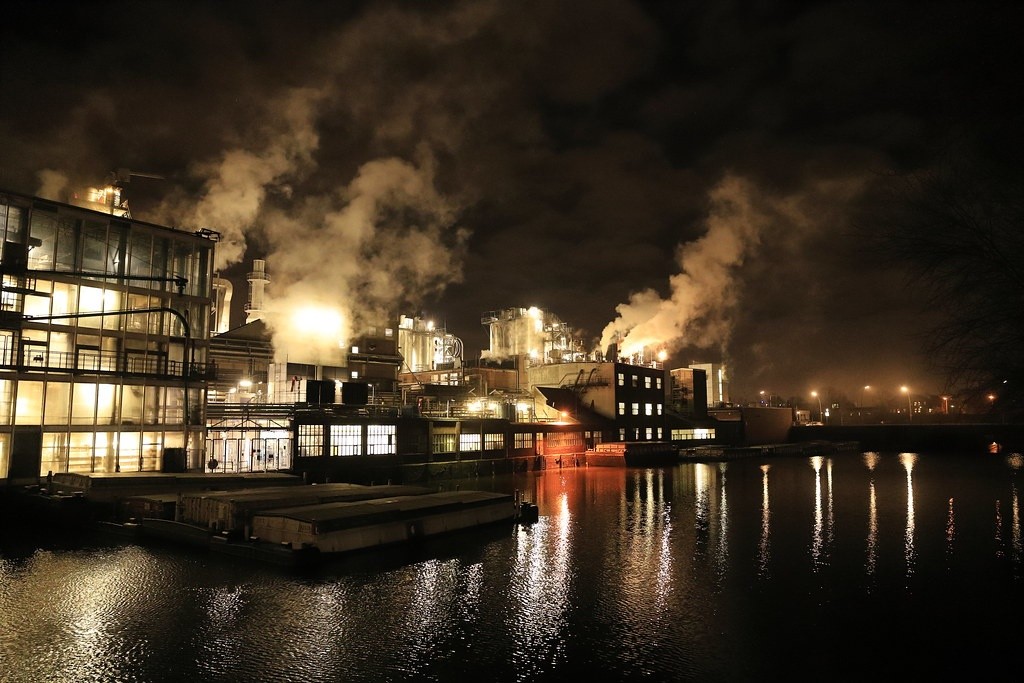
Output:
[861,385,871,426]
[810,389,822,423]
[899,385,912,425]
[943,397,947,414]
[988,396,994,406]
